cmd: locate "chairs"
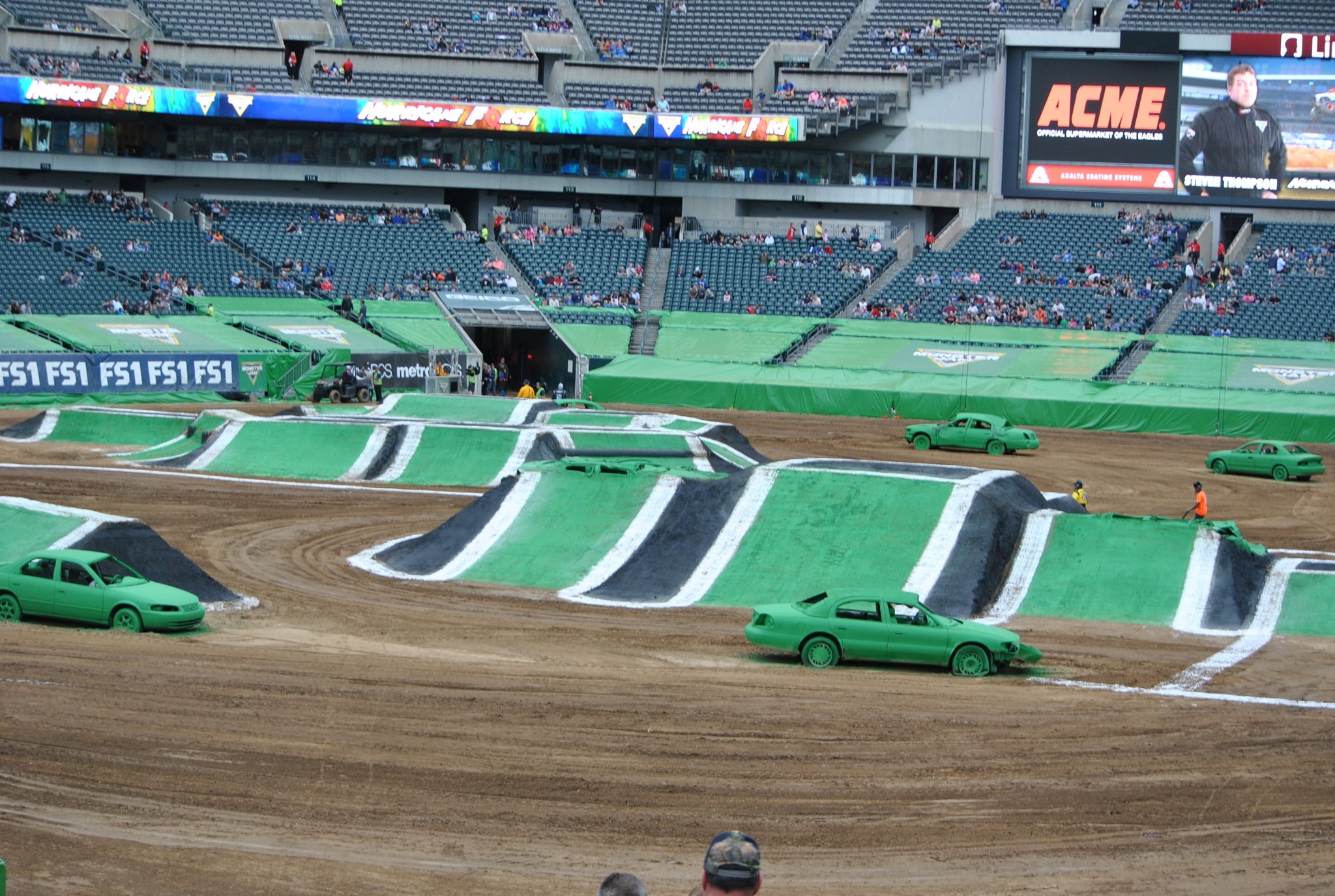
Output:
[1264,448,1271,454]
[0,179,1335,362]
[1117,1,1334,42]
[66,569,89,586]
[888,604,899,624]
[0,1,1065,133]
[975,420,984,429]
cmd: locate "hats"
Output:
[1072,480,1082,485]
[703,830,762,879]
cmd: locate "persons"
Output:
[1036,1,1271,12]
[975,420,985,428]
[1071,480,1088,510]
[598,870,648,896]
[688,829,764,896]
[1186,480,1209,520]
[24,0,1003,113]
[1178,62,1289,200]
[340,353,592,409]
[0,182,1334,346]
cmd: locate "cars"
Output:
[1203,436,1327,482]
[0,547,205,635]
[745,589,1042,682]
[518,457,728,485]
[549,397,605,412]
[899,410,1039,455]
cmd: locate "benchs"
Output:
[22,561,54,579]
